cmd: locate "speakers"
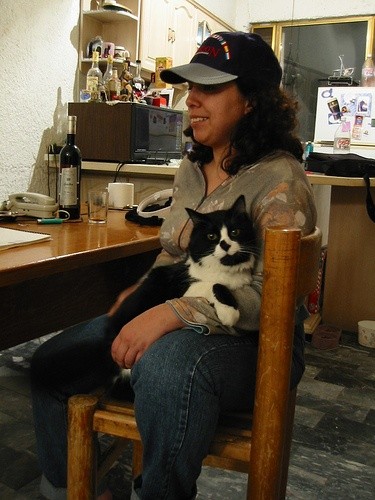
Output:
[66,102,183,164]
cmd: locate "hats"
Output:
[159,31,282,88]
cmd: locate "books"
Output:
[0,227,53,250]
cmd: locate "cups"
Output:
[91,91,100,102]
[101,42,115,59]
[114,46,130,60]
[87,189,109,224]
[327,98,341,120]
[80,89,91,102]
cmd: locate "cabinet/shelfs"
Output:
[77,0,142,76]
[139,0,198,74]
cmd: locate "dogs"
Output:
[102,189,267,407]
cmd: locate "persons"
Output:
[27,31,316,500]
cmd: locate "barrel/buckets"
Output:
[358,320,375,349]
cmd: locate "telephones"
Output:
[8,192,58,218]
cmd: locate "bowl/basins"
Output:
[311,324,341,350]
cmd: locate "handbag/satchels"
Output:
[303,151,375,178]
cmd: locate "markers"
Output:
[37,219,64,224]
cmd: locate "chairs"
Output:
[67,226,322,500]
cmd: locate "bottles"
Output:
[58,116,81,220]
[304,140,313,171]
[333,116,350,154]
[86,51,145,103]
[361,55,375,87]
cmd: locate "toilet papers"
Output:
[108,182,134,209]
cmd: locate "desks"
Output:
[0,199,178,358]
[58,162,375,331]
